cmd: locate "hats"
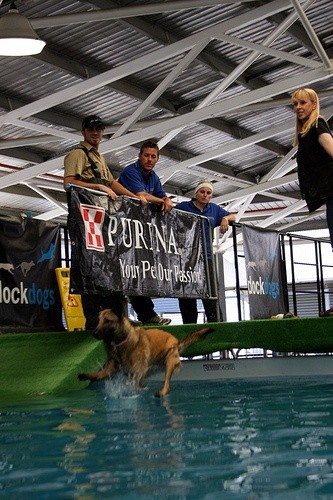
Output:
[82,114,105,129]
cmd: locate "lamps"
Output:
[0,0,46,56]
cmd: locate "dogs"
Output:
[78,295,216,397]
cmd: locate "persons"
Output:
[64,114,148,330]
[171,180,235,324]
[117,141,173,325]
[291,88,333,317]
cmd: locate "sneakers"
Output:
[143,315,171,325]
[127,318,143,326]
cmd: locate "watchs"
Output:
[223,217,227,220]
